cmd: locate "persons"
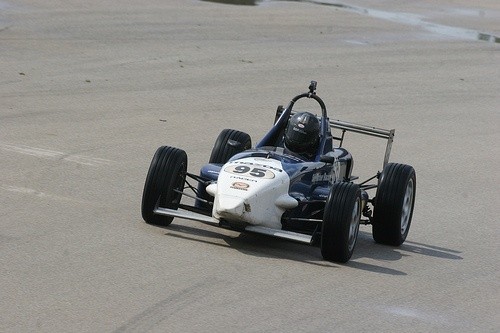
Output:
[281,111,321,159]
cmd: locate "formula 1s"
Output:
[142,80,416,263]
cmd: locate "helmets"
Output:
[284,112,320,156]
